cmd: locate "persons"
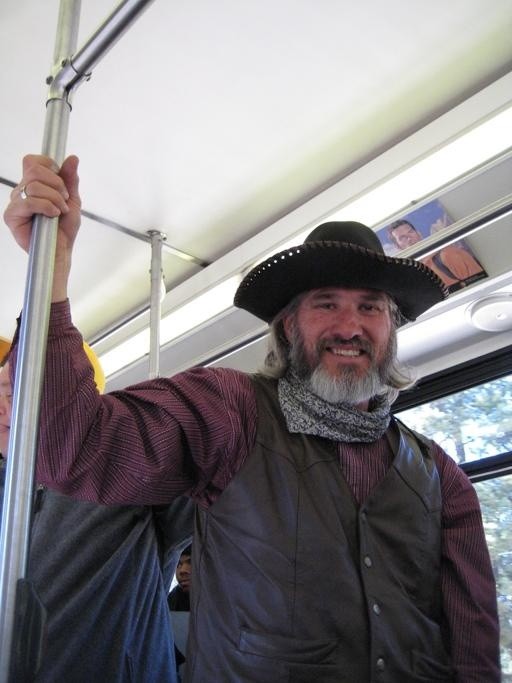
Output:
[166,542,192,682]
[387,217,482,288]
[1,151,501,683]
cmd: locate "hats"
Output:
[234,221,450,330]
[0,336,106,395]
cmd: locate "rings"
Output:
[17,181,27,199]
